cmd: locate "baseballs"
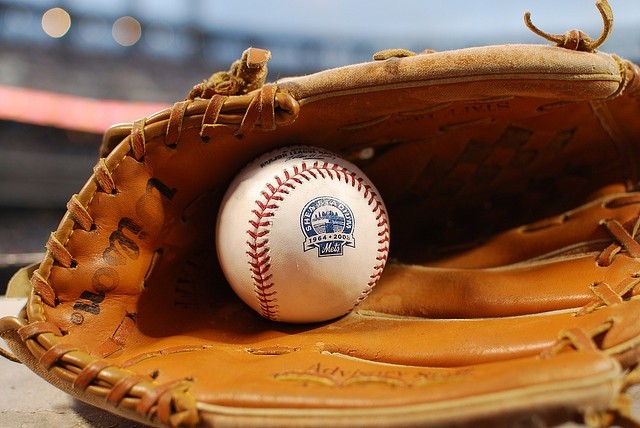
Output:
[215,145,390,323]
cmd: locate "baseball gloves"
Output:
[0,0,640,428]
